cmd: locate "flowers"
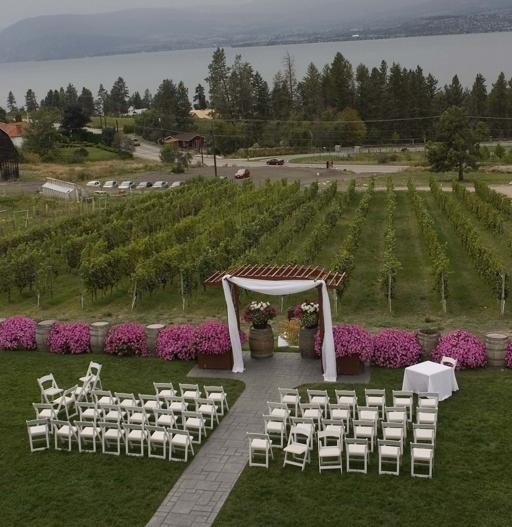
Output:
[244,301,276,324]
[314,325,374,361]
[188,320,247,356]
[294,300,319,328]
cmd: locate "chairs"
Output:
[26,361,229,462]
[442,357,459,390]
[246,387,440,479]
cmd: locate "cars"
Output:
[103,180,117,190]
[152,181,169,188]
[86,181,101,188]
[234,169,250,179]
[170,181,185,189]
[118,180,135,189]
[135,182,152,190]
[267,159,284,165]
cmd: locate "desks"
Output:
[401,361,457,402]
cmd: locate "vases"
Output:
[197,351,233,369]
[304,319,318,329]
[251,320,266,328]
[320,354,364,375]
